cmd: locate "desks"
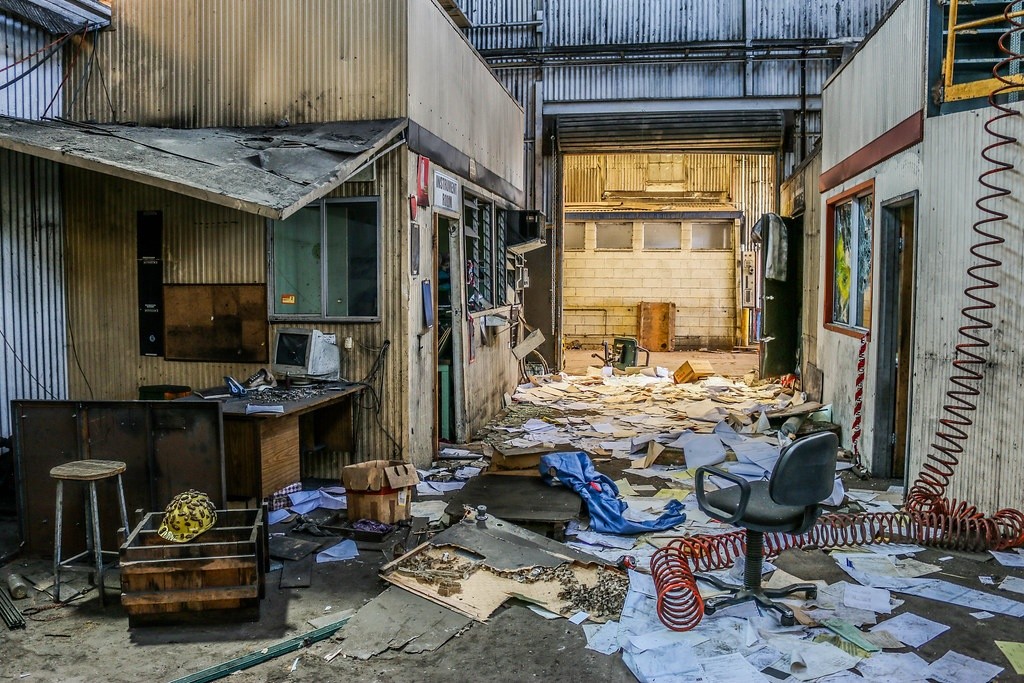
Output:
[170,383,367,506]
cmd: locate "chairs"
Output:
[592,336,651,371]
[695,430,838,625]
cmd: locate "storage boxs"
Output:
[341,459,419,525]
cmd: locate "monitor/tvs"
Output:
[272,328,340,385]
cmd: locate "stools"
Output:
[49,458,130,610]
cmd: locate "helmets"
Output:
[158,489,218,543]
